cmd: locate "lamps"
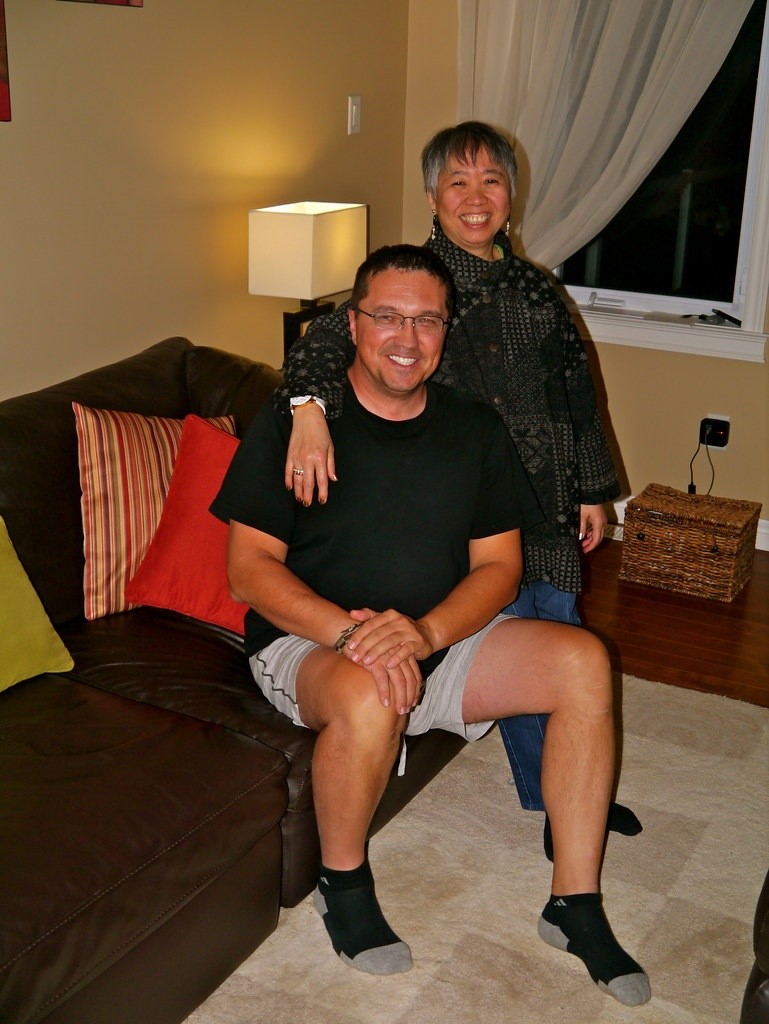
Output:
[248,201,367,360]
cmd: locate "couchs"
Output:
[0,338,469,1024]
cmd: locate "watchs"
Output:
[290,395,326,416]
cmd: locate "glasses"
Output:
[353,308,448,334]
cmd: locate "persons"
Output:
[271,120,643,863]
[208,244,652,1007]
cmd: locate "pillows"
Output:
[71,400,238,619]
[127,414,252,636]
[0,513,76,696]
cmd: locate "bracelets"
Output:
[333,621,373,655]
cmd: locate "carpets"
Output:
[179,670,769,1024]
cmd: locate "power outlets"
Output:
[704,413,730,451]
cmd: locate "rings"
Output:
[293,469,305,476]
